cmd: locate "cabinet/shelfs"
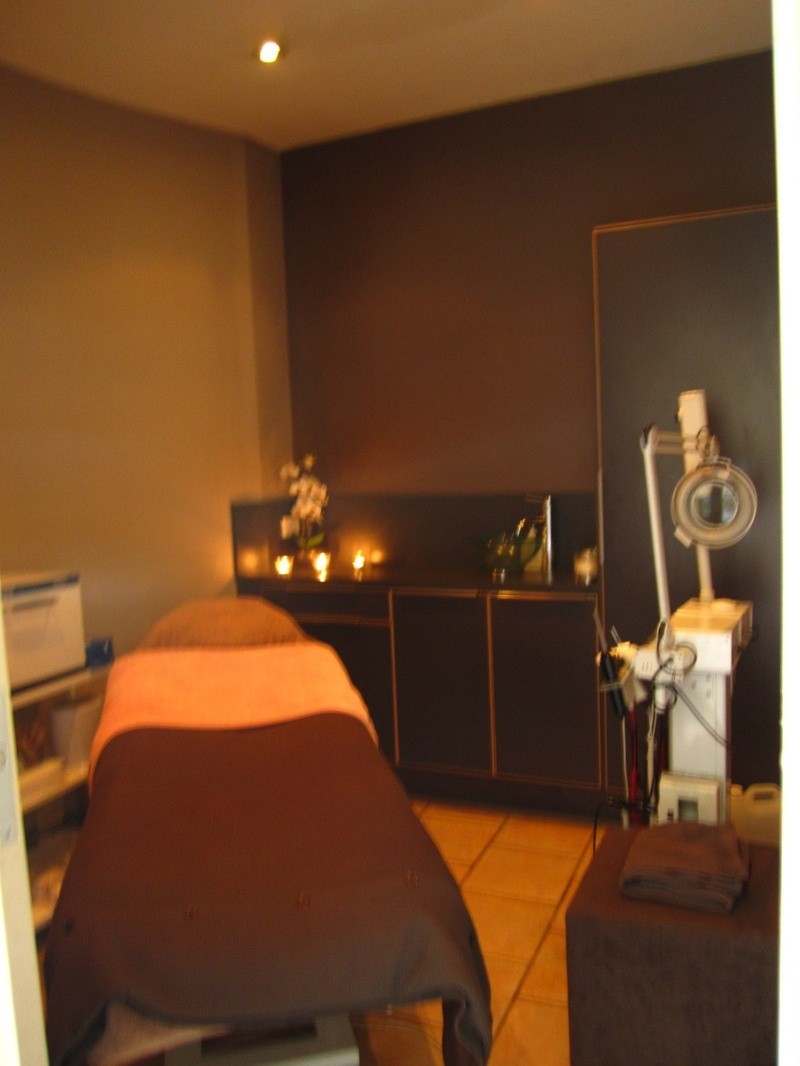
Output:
[230,488,609,820]
[1,637,117,1066]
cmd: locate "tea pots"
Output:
[485,531,522,570]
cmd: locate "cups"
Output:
[352,550,366,571]
[311,552,332,575]
[277,555,295,576]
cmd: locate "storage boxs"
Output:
[1,563,88,692]
